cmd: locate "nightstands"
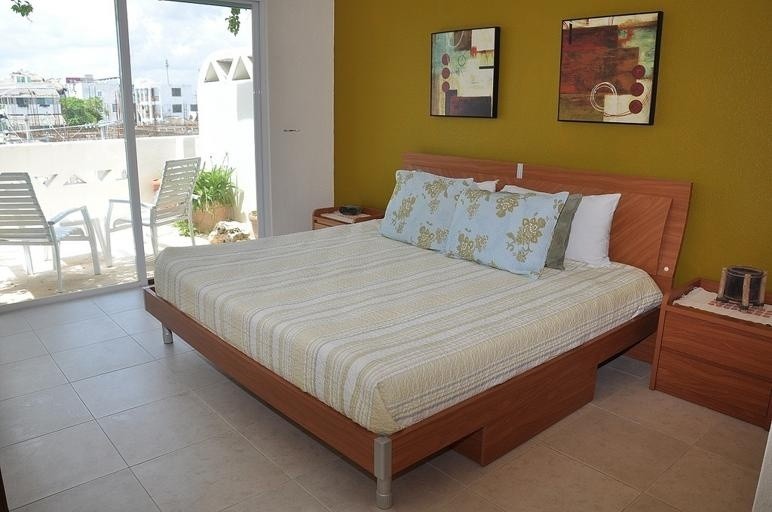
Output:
[649,276,772,431]
[310,205,384,233]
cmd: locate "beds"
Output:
[143,150,694,509]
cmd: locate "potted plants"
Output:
[184,152,240,235]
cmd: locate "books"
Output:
[320,209,372,225]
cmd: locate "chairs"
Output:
[104,157,202,267]
[0,170,101,294]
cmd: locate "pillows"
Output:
[378,170,624,280]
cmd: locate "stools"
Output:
[43,214,106,270]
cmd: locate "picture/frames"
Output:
[557,11,663,126]
[429,26,501,119]
